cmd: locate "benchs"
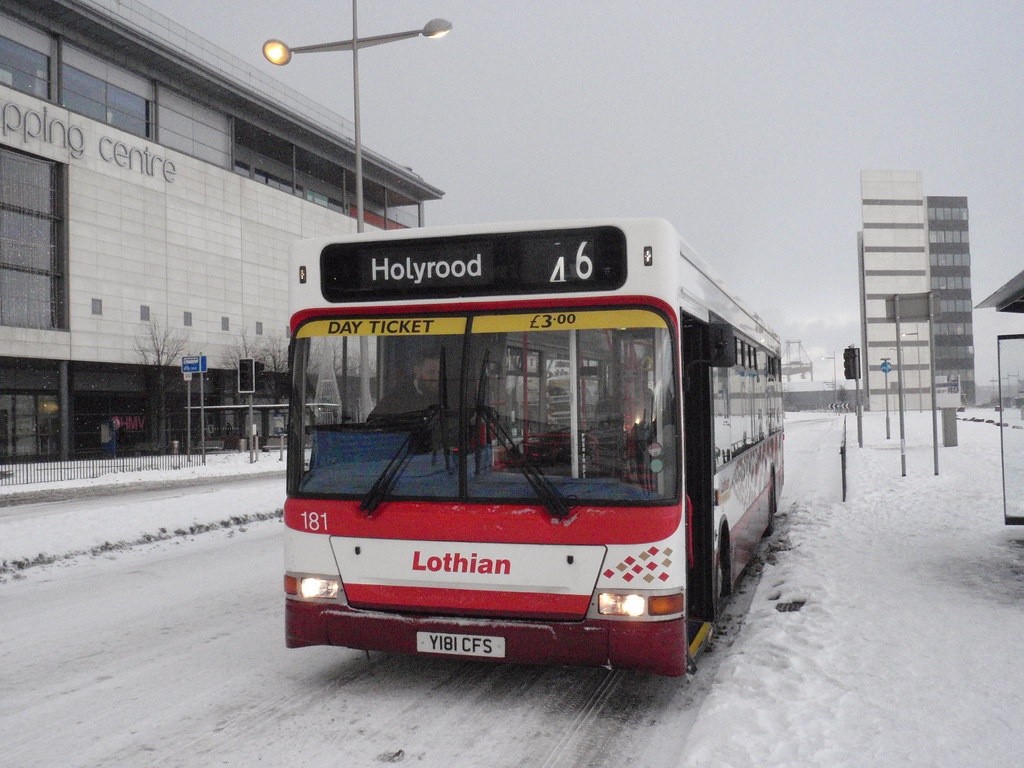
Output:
[134,442,156,457]
[192,441,224,456]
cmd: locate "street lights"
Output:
[889,345,906,412]
[260,1,453,424]
[820,351,838,412]
[900,323,923,413]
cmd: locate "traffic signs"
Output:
[181,355,207,373]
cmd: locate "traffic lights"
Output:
[239,359,255,394]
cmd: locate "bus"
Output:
[281,210,791,681]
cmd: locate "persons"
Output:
[365,347,454,422]
[226,423,232,436]
